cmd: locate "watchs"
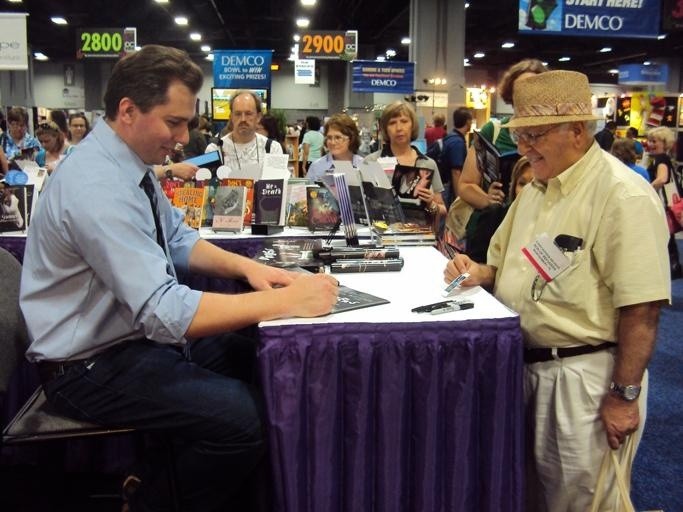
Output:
[609,381,642,402]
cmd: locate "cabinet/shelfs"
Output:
[281,134,299,179]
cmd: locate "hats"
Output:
[496,69,608,130]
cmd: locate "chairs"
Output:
[0,243,201,512]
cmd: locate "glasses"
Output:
[325,134,350,144]
[507,123,565,144]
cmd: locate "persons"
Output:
[18,44,339,512]
[2,60,682,256]
[444,71,674,512]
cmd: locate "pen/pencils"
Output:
[445,243,458,260]
[297,267,314,275]
[411,300,474,315]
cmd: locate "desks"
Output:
[0,218,439,295]
[252,242,527,512]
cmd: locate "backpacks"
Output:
[425,134,464,185]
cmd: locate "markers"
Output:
[318,245,404,273]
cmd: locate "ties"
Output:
[139,172,167,257]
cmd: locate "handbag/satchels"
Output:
[653,159,683,239]
[442,189,477,240]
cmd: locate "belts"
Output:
[525,343,616,365]
